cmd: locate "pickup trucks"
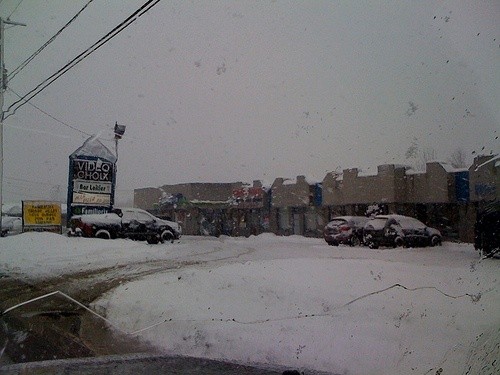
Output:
[70,207,182,245]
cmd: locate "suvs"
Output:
[324,215,369,248]
[362,213,443,249]
